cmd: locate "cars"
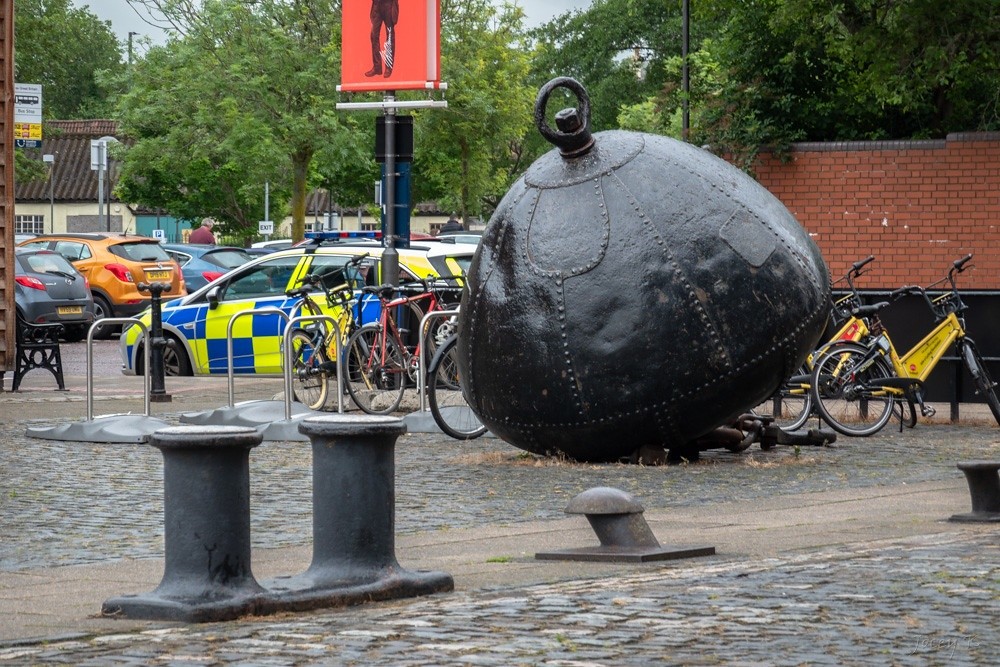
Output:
[13,234,49,247]
[11,247,97,346]
[239,228,489,261]
[14,232,189,341]
[123,242,274,305]
[120,230,479,376]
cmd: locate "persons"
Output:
[188,218,216,244]
[439,214,465,232]
[365,0,399,78]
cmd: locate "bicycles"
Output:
[339,274,462,416]
[809,253,1000,436]
[745,254,920,433]
[278,252,379,412]
[427,277,490,441]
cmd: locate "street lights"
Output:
[42,155,55,232]
